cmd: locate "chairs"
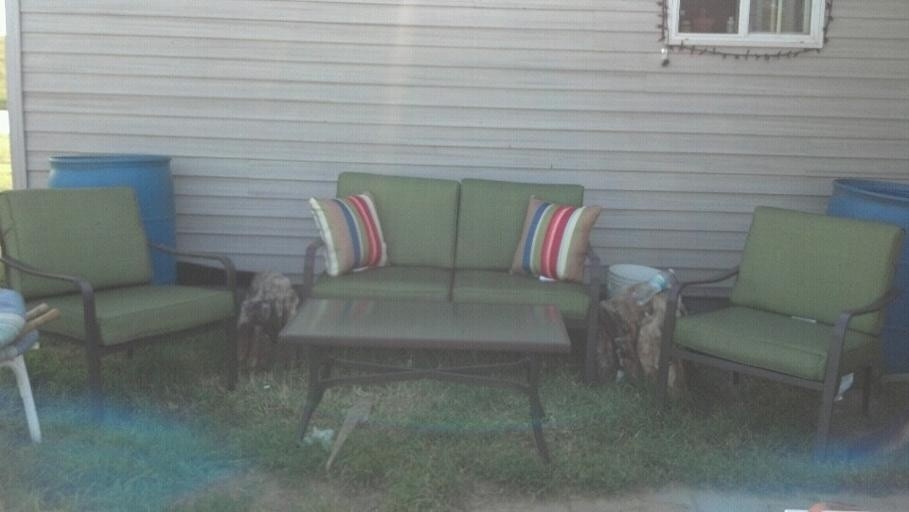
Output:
[654,207,905,464]
[0,186,241,428]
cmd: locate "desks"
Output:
[276,295,572,466]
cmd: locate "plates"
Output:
[604,264,658,297]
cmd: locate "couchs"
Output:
[302,169,603,386]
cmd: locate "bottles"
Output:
[629,265,679,308]
[726,15,735,33]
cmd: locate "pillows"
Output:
[305,190,388,278]
[508,194,601,283]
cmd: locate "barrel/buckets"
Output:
[604,263,662,299]
[48,151,181,287]
[826,176,909,375]
